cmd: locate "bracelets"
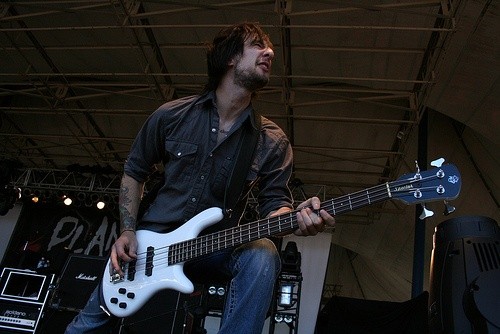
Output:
[120,230,136,236]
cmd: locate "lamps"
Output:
[3,186,120,213]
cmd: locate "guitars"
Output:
[101,155,463,318]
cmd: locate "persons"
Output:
[64,23,336,334]
